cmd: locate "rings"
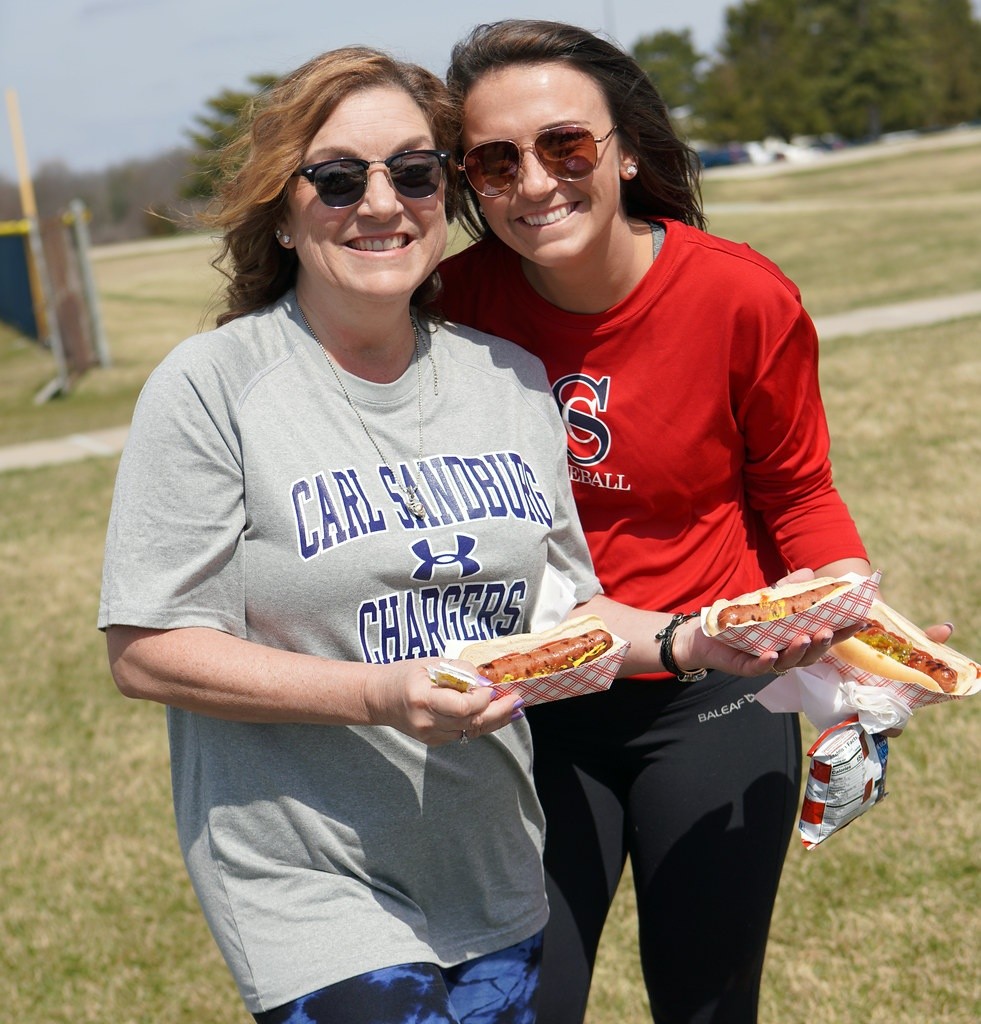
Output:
[459,730,470,746]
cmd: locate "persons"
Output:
[95,43,866,1024]
[442,19,959,1024]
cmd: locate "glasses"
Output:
[457,123,618,198]
[291,150,451,209]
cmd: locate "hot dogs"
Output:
[706,577,864,636]
[459,614,623,685]
[829,617,978,696]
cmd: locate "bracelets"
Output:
[656,609,708,684]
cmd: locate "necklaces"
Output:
[289,290,438,523]
